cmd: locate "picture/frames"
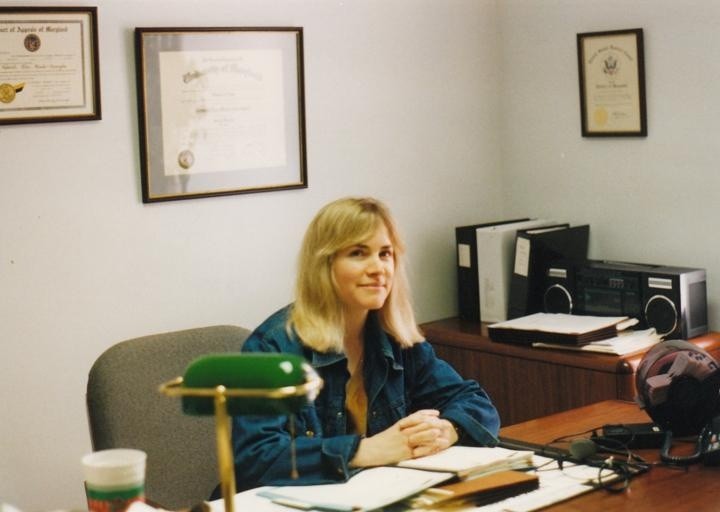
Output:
[0,5,103,124]
[576,29,648,138]
[132,26,308,205]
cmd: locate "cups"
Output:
[81,448,148,511]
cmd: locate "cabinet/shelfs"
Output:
[418,315,720,424]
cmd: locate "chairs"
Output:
[84,326,253,512]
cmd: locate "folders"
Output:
[455,217,590,322]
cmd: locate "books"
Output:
[484,311,665,358]
[404,470,542,511]
[265,437,537,511]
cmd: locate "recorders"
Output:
[542,258,707,340]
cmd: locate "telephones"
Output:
[702,417,720,466]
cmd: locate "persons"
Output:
[209,195,503,498]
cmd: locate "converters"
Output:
[603,423,666,449]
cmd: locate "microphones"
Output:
[569,437,617,460]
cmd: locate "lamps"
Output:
[159,353,324,512]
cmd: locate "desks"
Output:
[497,398,720,512]
